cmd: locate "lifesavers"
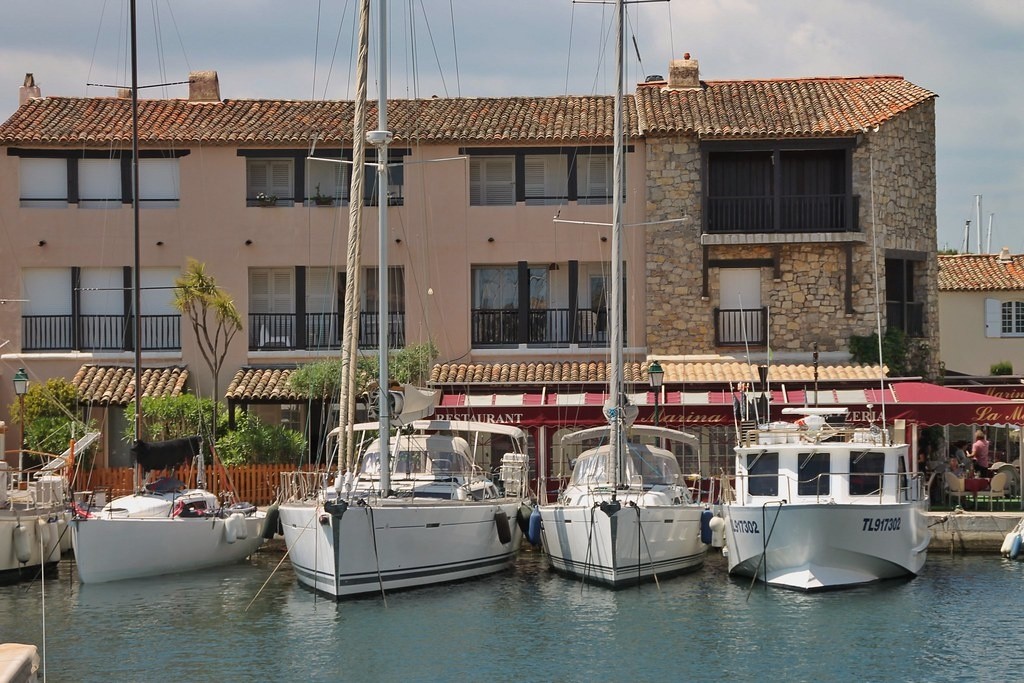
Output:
[794,415,826,426]
[70,502,93,518]
[169,502,185,518]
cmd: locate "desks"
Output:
[966,479,989,511]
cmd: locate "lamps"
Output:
[156,241,164,246]
[396,238,402,244]
[37,240,48,247]
[549,263,560,271]
[488,237,494,243]
[600,236,608,241]
[245,239,253,246]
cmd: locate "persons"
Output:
[918,431,990,479]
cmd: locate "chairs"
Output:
[944,472,1006,512]
[578,310,597,343]
[259,325,292,352]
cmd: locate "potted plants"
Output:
[310,183,333,205]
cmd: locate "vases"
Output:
[260,201,274,206]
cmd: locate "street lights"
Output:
[13,368,30,483]
[647,359,664,448]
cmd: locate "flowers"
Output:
[256,191,279,203]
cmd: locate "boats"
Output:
[0,450,74,585]
[709,407,933,591]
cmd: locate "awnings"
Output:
[431,382,1024,427]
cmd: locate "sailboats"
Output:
[531,1,707,584]
[71,0,277,587]
[275,0,533,603]
